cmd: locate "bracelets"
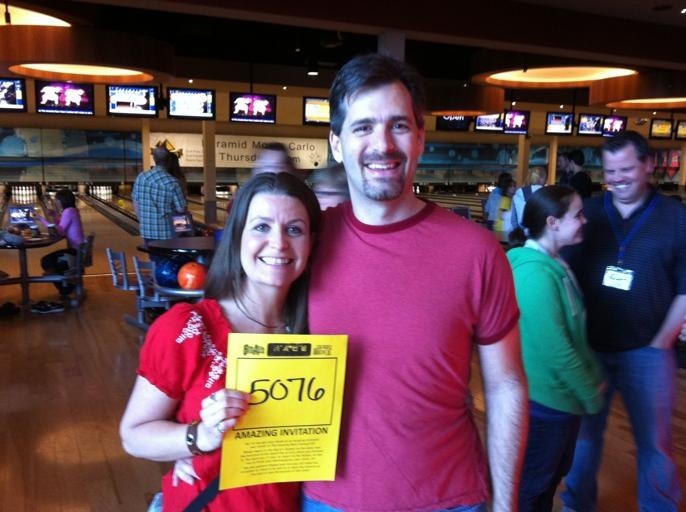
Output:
[186,422,211,455]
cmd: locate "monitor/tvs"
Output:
[650,118,673,138]
[476,110,504,133]
[605,114,626,136]
[229,91,276,123]
[545,112,574,134]
[167,87,216,120]
[8,206,36,224]
[504,109,529,134]
[675,119,686,138]
[172,212,196,237]
[106,83,159,119]
[578,112,604,135]
[303,96,331,125]
[0,77,26,113]
[34,80,95,116]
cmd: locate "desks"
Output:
[147,237,219,266]
[0,228,62,306]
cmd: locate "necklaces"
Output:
[231,287,291,333]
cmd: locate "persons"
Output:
[131,146,188,267]
[485,174,513,229]
[116,174,322,511]
[512,166,548,232]
[491,180,518,230]
[570,149,595,201]
[292,56,530,510]
[303,166,351,213]
[505,186,611,511]
[165,151,188,205]
[560,131,686,512]
[252,141,300,173]
[556,151,571,186]
[31,188,82,314]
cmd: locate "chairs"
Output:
[108,245,151,323]
[30,234,99,307]
[131,258,182,312]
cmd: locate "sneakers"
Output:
[30,301,65,314]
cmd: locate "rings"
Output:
[216,424,225,433]
[210,392,218,402]
